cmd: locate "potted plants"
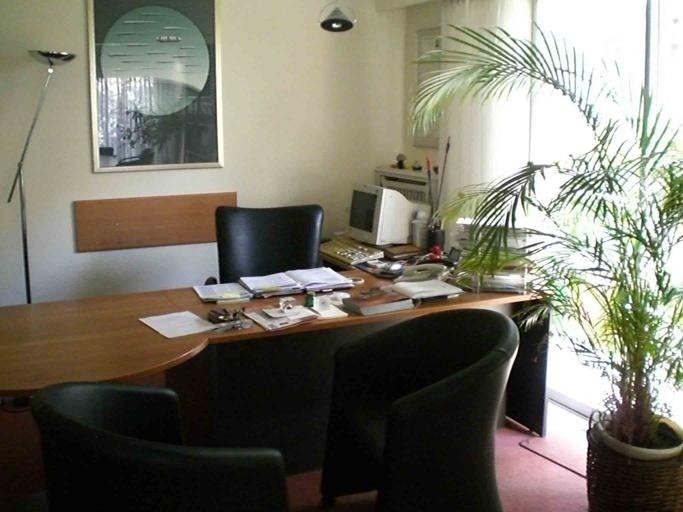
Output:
[401,2,680,510]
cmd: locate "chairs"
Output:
[26,372,296,512]
[203,202,326,289]
[323,307,519,512]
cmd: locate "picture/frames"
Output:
[82,1,228,176]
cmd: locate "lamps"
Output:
[1,46,81,305]
[315,0,357,37]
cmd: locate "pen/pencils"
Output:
[321,287,353,293]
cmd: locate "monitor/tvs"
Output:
[346,182,432,247]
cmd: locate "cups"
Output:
[279,297,294,312]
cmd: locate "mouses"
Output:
[389,263,401,271]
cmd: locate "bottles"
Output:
[304,291,315,307]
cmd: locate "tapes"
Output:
[349,277,364,285]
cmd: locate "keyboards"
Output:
[319,238,384,266]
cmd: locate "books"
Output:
[385,244,421,260]
[341,291,413,316]
[391,279,463,300]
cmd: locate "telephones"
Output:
[392,258,455,284]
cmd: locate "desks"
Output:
[0,234,556,440]
